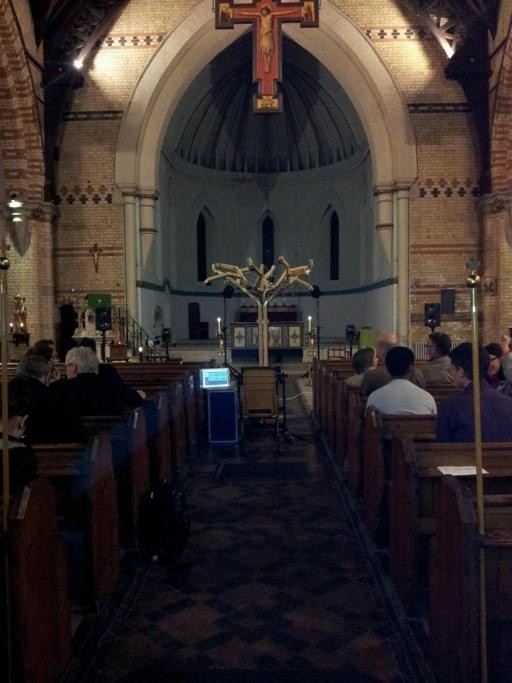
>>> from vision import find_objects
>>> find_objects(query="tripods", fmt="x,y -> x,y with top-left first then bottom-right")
222,302 -> 241,377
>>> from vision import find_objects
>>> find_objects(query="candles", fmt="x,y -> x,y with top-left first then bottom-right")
217,317 -> 222,335
308,315 -> 312,332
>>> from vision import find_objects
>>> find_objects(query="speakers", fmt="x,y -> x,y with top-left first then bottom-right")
162,327 -> 171,344
424,303 -> 441,328
345,324 -> 356,339
441,289 -> 455,311
96,306 -> 113,329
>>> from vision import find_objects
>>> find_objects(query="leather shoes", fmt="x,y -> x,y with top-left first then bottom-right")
117,549 -> 159,577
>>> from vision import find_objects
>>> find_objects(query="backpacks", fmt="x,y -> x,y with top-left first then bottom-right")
138,477 -> 192,564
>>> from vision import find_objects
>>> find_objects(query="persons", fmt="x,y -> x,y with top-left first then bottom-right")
345,330 -> 512,495
236,5 -> 300,74
1,330 -> 151,504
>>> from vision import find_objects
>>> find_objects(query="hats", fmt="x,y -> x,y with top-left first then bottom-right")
351,346 -> 377,376
